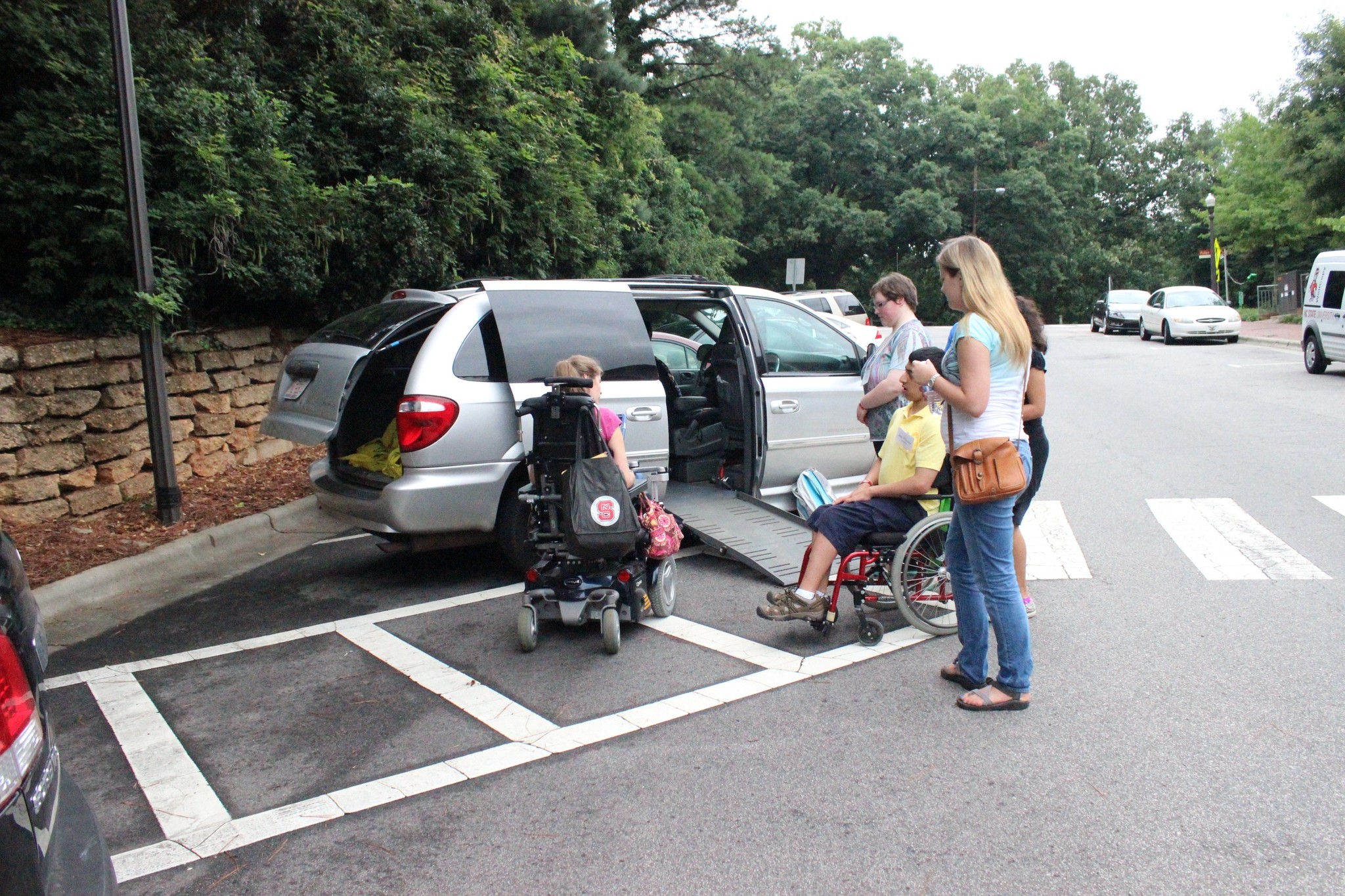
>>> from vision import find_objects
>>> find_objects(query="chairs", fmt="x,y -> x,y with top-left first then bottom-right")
653,356 -> 707,409
709,359 -> 745,465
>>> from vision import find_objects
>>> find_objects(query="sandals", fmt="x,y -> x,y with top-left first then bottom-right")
940,658 -> 986,691
956,677 -> 1029,711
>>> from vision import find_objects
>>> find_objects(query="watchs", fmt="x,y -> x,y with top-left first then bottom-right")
928,373 -> 941,387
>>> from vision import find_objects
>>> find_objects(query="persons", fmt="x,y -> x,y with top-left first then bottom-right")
529,354 -> 642,490
914,236 -> 1033,710
983,296 -> 1048,622
757,347 -> 949,621
856,274 -> 932,455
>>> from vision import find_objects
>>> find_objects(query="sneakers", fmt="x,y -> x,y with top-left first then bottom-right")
766,586 -> 830,619
756,590 -> 823,621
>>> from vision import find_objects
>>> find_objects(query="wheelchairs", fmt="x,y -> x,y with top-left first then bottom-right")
802,493 -> 959,647
513,375 -> 678,655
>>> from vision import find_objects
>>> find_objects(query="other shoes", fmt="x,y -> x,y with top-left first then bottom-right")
988,600 -> 1036,622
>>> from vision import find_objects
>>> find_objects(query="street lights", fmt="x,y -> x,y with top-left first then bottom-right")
1205,192 -> 1218,293
972,165 -> 1006,237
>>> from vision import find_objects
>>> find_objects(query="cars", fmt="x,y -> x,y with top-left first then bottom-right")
1138,285 -> 1241,345
0,532 -> 120,896
1090,289 -> 1152,335
765,311 -> 893,353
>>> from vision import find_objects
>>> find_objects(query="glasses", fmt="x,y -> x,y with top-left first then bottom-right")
873,298 -> 892,310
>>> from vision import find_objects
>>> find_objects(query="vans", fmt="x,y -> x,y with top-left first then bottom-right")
1300,249 -> 1345,374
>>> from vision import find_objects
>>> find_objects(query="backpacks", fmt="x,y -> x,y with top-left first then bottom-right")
791,468 -> 838,521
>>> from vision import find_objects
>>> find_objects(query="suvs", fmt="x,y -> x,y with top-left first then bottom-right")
762,289 -> 871,326
258,277 -> 877,582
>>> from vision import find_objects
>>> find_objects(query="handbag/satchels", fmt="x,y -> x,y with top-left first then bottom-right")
559,406 -> 641,558
637,491 -> 683,558
953,436 -> 1025,503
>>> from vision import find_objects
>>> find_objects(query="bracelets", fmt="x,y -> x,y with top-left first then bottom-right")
859,403 -> 868,410
